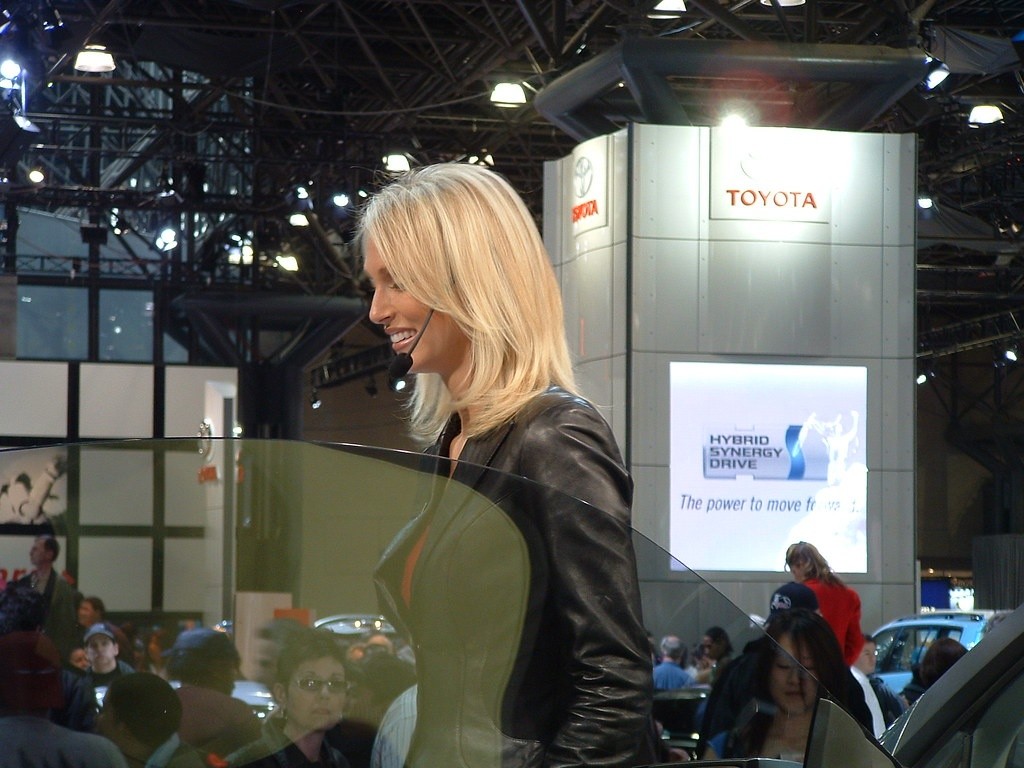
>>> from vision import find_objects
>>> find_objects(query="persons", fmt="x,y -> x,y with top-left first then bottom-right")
786,542 -> 863,666
696,582 -> 821,757
851,635 -> 905,738
360,163 -> 655,768
649,626 -> 734,735
707,607 -> 844,768
0,535 -> 417,768
921,638 -> 967,688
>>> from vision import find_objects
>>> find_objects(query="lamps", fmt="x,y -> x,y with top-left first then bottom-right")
490,58 -> 527,108
72,33 -> 116,73
969,105 -> 1005,129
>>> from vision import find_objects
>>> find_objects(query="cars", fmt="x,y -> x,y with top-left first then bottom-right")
1,434 -> 1023,768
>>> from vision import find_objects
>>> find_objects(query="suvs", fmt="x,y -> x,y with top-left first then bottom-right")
860,606 -> 1016,694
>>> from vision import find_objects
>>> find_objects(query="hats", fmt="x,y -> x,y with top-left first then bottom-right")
769,581 -> 820,614
0,630 -> 61,676
82,624 -> 115,643
159,626 -> 248,684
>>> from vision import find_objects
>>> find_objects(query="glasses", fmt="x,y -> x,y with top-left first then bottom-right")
290,677 -> 348,694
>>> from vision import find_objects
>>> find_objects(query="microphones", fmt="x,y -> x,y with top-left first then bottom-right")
389,309 -> 434,379
